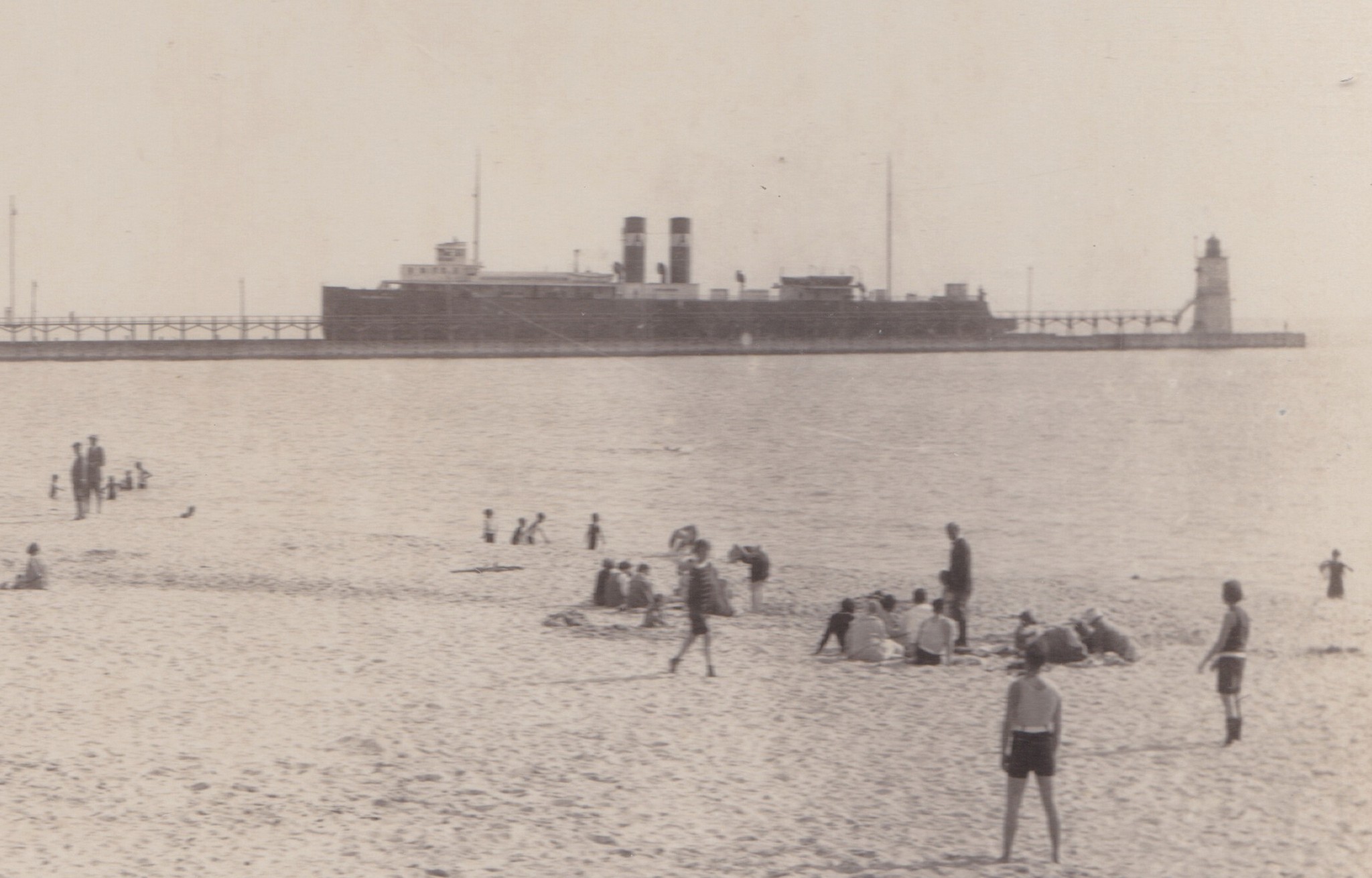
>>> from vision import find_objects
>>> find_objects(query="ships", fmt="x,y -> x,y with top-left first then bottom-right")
320,140 -> 1018,343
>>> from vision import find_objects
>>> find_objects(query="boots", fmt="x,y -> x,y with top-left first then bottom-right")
1225,717 -> 1241,744
707,665 -> 717,677
671,656 -> 684,673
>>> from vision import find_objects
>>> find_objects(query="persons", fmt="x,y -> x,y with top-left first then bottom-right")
1318,549 -> 1353,600
478,492 -> 1134,674
995,647 -> 1069,867
47,429 -> 156,517
2,540 -> 48,590
1196,579 -> 1252,745
